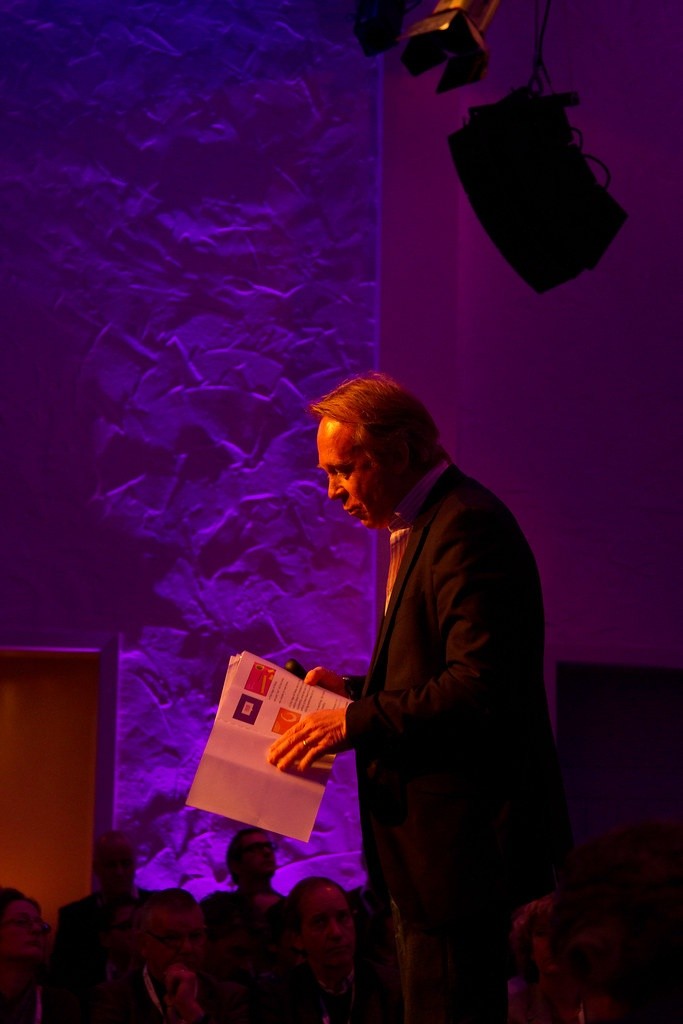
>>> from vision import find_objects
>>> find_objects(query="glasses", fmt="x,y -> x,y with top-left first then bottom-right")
3,917 -> 51,932
302,912 -> 356,925
240,843 -> 277,851
147,931 -> 206,944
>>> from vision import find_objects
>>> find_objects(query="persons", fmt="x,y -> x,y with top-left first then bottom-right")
0,878 -> 683,1024
267,372 -> 578,1024
51,832 -> 168,1010
599,817 -> 683,908
200,827 -> 292,959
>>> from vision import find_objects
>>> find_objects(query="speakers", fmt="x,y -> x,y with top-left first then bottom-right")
448,85 -> 628,295
